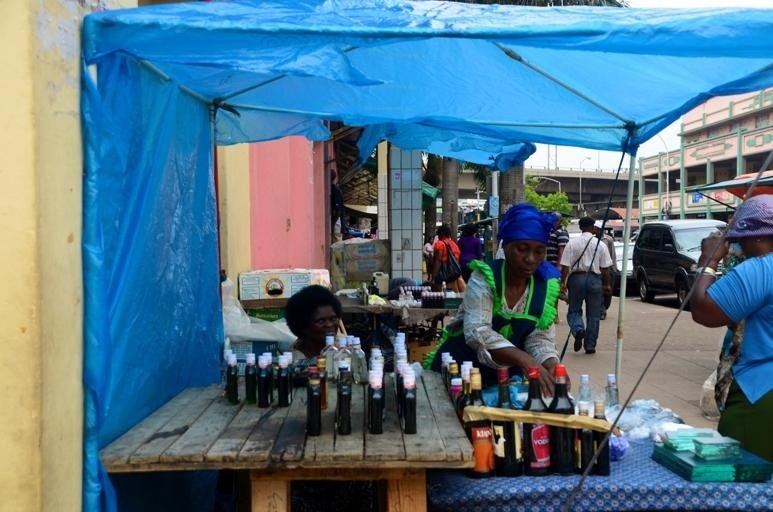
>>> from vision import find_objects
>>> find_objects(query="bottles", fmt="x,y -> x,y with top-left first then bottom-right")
439,351 -> 619,477
222,331 -> 418,436
403,283 -> 466,308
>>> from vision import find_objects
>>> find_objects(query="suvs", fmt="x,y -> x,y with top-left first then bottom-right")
632,218 -> 741,307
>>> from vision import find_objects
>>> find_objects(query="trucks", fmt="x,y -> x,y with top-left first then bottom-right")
562,217 -> 592,245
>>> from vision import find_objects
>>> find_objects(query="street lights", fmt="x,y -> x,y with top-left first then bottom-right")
532,176 -> 562,192
579,157 -> 592,208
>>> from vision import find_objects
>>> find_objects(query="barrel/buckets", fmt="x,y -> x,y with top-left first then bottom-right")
371,272 -> 389,297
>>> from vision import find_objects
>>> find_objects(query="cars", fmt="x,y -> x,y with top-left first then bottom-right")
609,240 -> 638,295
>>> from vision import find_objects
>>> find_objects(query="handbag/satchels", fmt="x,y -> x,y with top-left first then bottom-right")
433,239 -> 462,285
698,352 -> 738,421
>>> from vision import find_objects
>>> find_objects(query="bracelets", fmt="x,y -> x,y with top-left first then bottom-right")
696,267 -> 717,277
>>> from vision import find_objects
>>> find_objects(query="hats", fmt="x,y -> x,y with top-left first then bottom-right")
578,217 -> 595,226
721,192 -> 773,241
496,202 -> 558,248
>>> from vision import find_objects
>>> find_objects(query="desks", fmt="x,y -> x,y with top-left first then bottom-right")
390,302 -> 457,325
239,297 -> 392,347
99,369 -> 476,512
427,400 -> 773,512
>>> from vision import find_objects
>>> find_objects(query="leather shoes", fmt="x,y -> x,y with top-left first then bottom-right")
585,349 -> 596,354
573,329 -> 586,353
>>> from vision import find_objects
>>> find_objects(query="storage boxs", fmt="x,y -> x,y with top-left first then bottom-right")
231,342 -> 277,377
344,240 -> 390,281
239,268 -> 328,300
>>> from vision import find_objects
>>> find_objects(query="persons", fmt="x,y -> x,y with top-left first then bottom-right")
559,217 -> 613,353
285,285 -> 346,363
547,211 -> 569,324
691,193 -> 773,461
592,221 -> 617,320
421,225 -> 484,292
330,168 -> 344,243
422,203 -> 571,398
